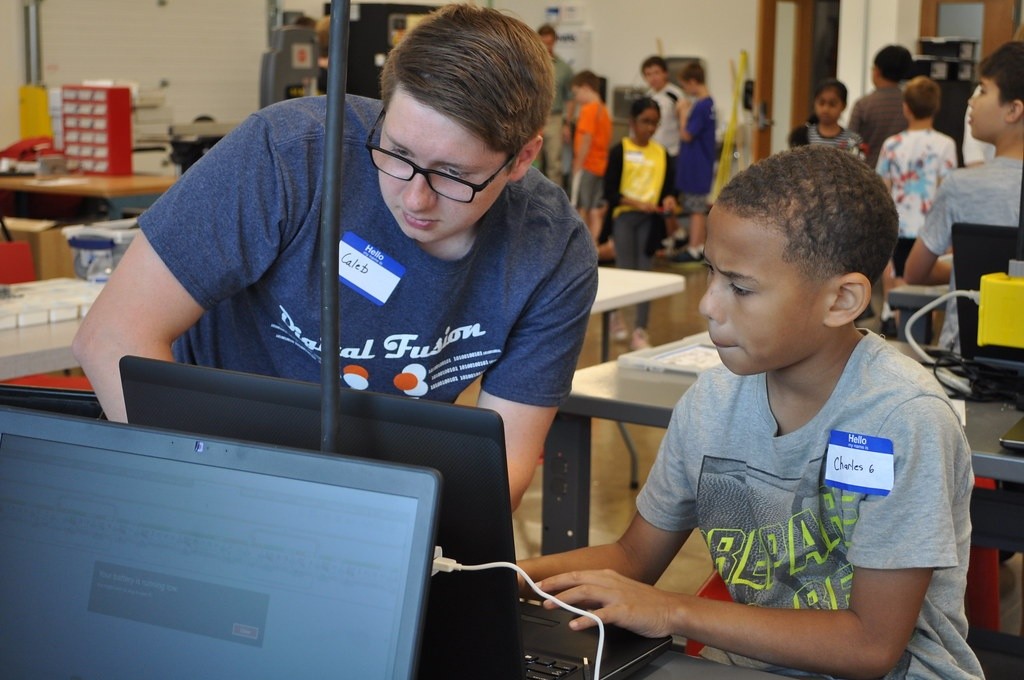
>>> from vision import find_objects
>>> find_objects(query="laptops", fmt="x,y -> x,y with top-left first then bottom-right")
119,355 -> 674,680
0,404 -> 443,680
951,220 -> 1024,378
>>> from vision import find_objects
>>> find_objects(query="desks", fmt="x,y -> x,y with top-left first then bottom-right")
0,175 -> 178,222
543,332 -> 1023,659
887,285 -> 949,345
590,266 -> 686,490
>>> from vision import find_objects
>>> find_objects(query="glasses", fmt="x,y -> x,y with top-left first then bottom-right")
633,118 -> 661,128
365,106 -> 520,204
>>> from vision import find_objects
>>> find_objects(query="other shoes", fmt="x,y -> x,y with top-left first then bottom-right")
669,248 -> 706,269
610,312 -> 628,343
630,330 -> 651,351
671,235 -> 689,249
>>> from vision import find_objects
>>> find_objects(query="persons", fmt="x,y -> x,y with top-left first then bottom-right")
72,3 -> 600,514
524,22 -> 718,355
517,143 -> 987,680
787,40 -> 1024,354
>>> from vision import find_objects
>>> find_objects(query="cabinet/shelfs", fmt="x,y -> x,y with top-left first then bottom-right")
61,85 -> 131,178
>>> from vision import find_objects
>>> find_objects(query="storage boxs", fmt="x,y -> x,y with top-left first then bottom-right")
0,215 -> 74,281
